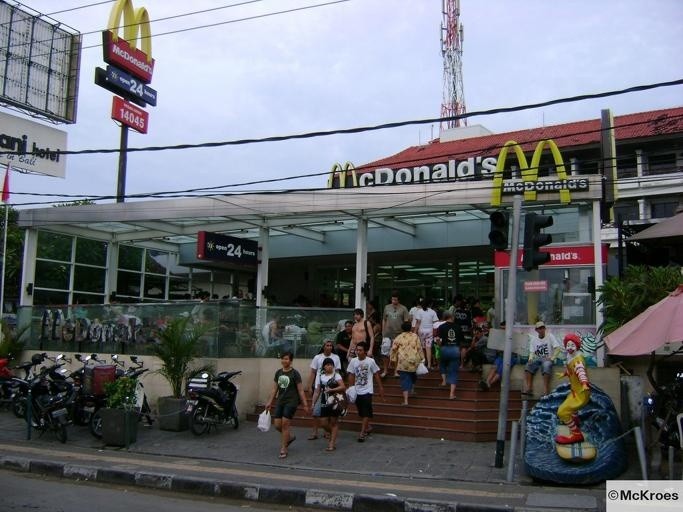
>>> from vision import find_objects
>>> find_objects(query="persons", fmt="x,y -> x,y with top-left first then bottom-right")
520,320 -> 560,396
480,321 -> 517,391
555,333 -> 591,444
71,289 -> 497,460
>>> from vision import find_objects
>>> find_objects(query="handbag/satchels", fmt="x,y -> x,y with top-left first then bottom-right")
367,337 -> 377,353
311,393 -> 322,416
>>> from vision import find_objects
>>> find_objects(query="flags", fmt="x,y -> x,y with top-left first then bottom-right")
1,162 -> 12,202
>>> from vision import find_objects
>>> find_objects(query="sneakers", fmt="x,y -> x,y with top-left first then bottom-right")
480,379 -> 490,391
522,389 -> 533,396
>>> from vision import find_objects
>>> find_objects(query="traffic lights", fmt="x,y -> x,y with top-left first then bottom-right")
487,210 -> 509,251
521,212 -> 554,271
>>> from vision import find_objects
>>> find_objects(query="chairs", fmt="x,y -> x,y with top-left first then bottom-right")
211,318 -> 334,358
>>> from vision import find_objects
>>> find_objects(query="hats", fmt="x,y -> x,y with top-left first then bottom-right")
535,321 -> 545,329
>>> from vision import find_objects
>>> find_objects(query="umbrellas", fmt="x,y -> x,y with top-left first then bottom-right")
623,205 -> 683,241
603,283 -> 683,356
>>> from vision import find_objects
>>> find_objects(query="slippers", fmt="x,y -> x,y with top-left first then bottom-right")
287,436 -> 296,446
279,451 -> 288,458
326,447 -> 336,451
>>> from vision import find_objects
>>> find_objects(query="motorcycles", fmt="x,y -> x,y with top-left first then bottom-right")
184,368 -> 243,438
0,350 -> 154,443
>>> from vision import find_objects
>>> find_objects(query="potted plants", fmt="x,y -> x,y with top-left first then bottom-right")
99,377 -> 141,445
139,313 -> 211,433
